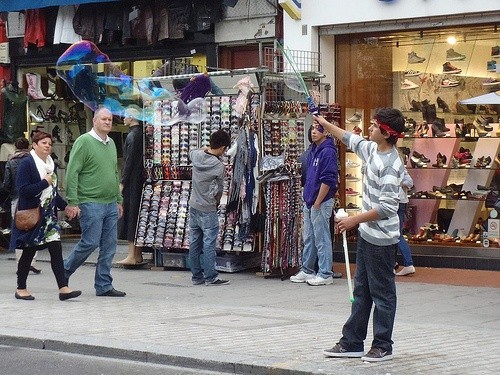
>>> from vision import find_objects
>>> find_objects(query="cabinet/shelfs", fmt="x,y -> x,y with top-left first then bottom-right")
346,107 -> 364,211
400,56 -> 500,249
29,97 -> 86,232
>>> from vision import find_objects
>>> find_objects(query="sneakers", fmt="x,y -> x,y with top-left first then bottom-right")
360,346 -> 393,362
441,49 -> 466,88
306,275 -> 334,286
323,342 -> 364,357
289,270 -> 316,283
483,45 -> 500,86
396,264 -> 415,276
193,278 -> 204,285
204,275 -> 231,287
400,52 -> 425,90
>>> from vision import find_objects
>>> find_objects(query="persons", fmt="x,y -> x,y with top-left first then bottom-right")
9,132 -> 82,300
188,130 -> 231,285
116,104 -> 143,264
63,107 -> 126,297
313,108 -> 415,362
290,121 -> 338,286
0,134 -> 29,249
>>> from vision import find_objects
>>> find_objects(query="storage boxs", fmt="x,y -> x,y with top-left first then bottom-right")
160,252 -> 186,268
199,253 -> 262,273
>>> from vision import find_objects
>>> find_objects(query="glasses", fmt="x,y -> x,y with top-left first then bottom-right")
135,95 -> 252,252
263,101 -> 310,176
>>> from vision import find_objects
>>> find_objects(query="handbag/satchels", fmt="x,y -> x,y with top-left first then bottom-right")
15,206 -> 41,231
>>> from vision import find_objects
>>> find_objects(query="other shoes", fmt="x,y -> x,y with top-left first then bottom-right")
59,290 -> 82,302
402,215 -> 491,244
401,96 -> 500,138
345,112 -> 363,211
28,265 -> 42,275
24,69 -> 84,168
407,183 -> 500,200
15,291 -> 36,300
398,147 -> 500,169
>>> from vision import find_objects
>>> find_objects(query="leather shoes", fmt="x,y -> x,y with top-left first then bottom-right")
96,287 -> 126,297
63,276 -> 69,288
